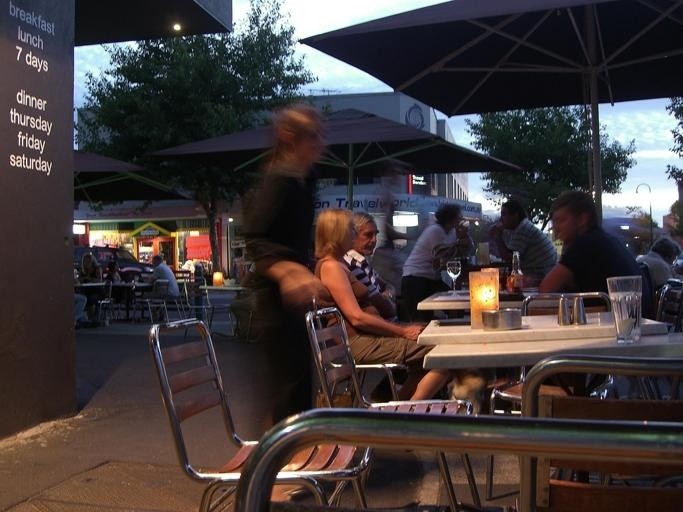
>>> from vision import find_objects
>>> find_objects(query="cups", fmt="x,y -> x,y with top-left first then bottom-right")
476,240 -> 490,265
605,275 -> 645,344
482,307 -> 521,331
213,271 -> 224,286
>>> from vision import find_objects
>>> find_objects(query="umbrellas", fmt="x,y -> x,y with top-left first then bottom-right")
297,0 -> 683,229
73,150 -> 192,202
143,108 -> 523,212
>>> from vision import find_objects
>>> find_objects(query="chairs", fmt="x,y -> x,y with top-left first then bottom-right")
518,354 -> 681,509
305,307 -> 479,509
647,278 -> 682,332
143,313 -> 371,511
74,248 -> 253,337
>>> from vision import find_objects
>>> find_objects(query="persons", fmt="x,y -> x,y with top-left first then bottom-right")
231,107 -> 330,442
75,295 -> 86,324
143,255 -> 180,322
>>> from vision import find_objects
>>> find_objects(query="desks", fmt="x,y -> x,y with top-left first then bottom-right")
419,328 -> 683,511
416,287 -> 576,305
418,314 -> 670,342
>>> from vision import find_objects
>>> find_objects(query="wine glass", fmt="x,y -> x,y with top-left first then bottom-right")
446,261 -> 462,297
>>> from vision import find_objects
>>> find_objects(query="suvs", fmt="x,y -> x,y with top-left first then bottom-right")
73,242 -> 154,284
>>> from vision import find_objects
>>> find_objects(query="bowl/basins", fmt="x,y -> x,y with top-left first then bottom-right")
223,279 -> 235,287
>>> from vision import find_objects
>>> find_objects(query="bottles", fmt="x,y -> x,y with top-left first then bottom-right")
504,251 -> 524,294
557,292 -> 586,325
140,247 -> 151,262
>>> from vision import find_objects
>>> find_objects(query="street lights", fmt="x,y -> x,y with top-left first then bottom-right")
225,217 -> 236,279
634,182 -> 652,245
